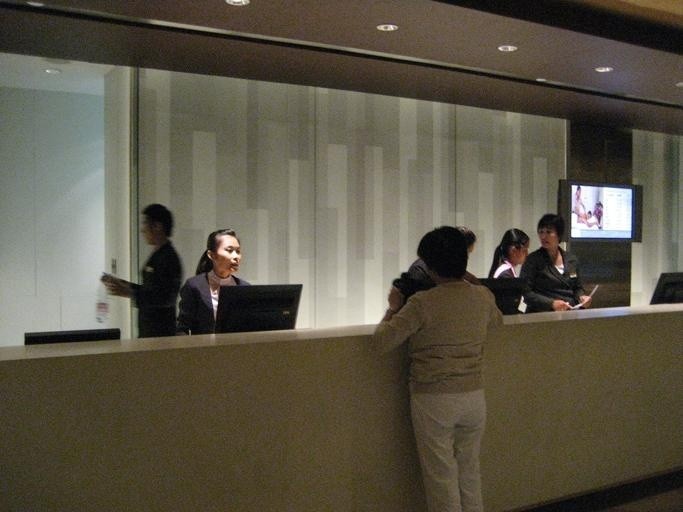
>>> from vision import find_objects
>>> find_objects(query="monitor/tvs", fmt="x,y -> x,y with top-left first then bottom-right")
479,275 -> 526,314
647,272 -> 683,306
24,327 -> 121,344
557,177 -> 644,246
214,283 -> 304,331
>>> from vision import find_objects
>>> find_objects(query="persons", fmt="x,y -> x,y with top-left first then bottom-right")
575,185 -> 604,229
371,223 -> 503,511
486,226 -> 531,315
381,226 -> 480,321
175,228 -> 253,337
518,212 -> 593,314
98,204 -> 181,336
